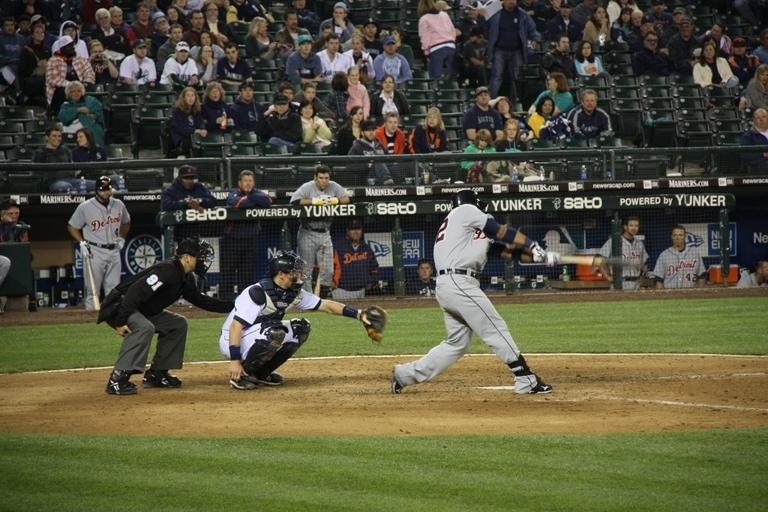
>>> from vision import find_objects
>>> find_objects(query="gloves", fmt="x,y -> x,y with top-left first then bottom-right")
528,238 -> 550,264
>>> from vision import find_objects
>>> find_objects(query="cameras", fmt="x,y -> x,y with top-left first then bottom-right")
90,52 -> 106,59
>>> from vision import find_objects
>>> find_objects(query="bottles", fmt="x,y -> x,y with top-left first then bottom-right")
538,165 -> 546,182
79,177 -> 86,192
405,163 -> 433,185
512,166 -> 518,183
550,171 -> 555,182
581,165 -> 587,179
221,111 -> 227,127
118,176 -> 125,189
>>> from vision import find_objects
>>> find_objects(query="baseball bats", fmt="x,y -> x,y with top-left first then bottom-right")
315,244 -> 326,296
87,257 -> 100,311
562,254 -> 637,271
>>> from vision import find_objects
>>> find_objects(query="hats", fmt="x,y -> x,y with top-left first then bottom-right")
1,199 -> 19,210
28,1 -> 748,133
178,165 -> 197,177
346,219 -> 363,230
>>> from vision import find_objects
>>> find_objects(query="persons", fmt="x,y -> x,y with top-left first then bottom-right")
95,236 -> 236,397
390,187 -> 554,395
1,1 -> 768,318
216,246 -> 389,390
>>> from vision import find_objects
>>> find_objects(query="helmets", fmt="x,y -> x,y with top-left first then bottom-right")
176,237 -> 215,278
452,189 -> 487,213
268,254 -> 308,285
95,176 -> 113,191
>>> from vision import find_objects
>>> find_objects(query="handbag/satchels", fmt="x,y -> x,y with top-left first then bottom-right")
550,118 -> 576,144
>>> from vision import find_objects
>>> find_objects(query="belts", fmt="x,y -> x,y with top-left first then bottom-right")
86,241 -> 115,250
439,267 -> 480,280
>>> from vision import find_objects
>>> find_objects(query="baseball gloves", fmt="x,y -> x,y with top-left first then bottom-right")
359,305 -> 386,342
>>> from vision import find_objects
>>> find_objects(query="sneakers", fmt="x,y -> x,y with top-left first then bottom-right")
229,376 -> 258,390
104,377 -> 138,395
392,365 -> 407,394
383,178 -> 395,186
28,302 -> 38,312
247,372 -> 284,386
142,369 -> 182,389
514,372 -> 553,395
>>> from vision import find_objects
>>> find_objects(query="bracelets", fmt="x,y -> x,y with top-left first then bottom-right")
229,346 -> 241,360
